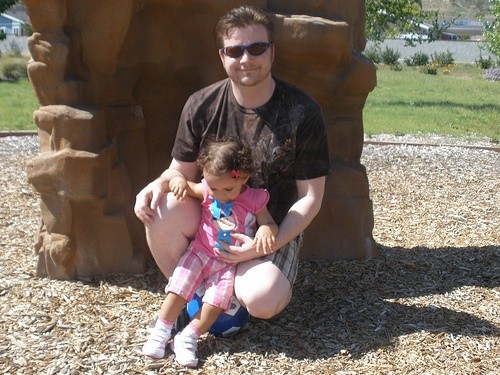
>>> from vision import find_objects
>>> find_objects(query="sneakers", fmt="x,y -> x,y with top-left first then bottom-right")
170,331 -> 199,368
142,323 -> 171,358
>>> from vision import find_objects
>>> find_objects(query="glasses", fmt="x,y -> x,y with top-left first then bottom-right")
217,40 -> 273,59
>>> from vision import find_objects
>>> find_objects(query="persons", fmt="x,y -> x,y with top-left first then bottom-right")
138,137 -> 284,368
133,3 -> 329,321
